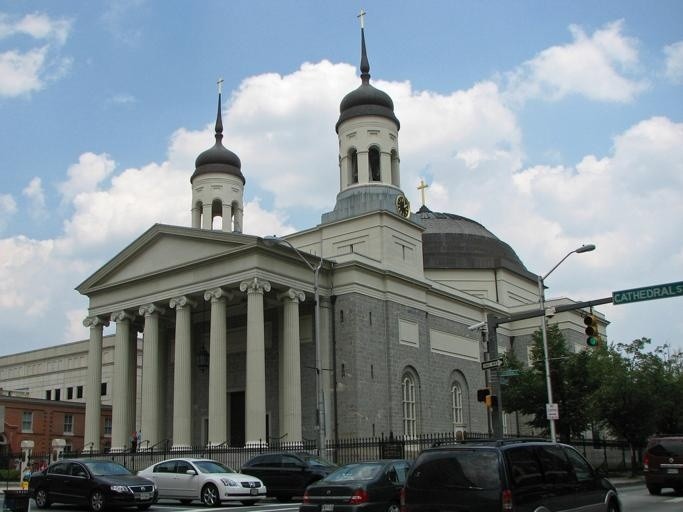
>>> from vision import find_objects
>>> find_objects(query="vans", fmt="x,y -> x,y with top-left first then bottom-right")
400,443 -> 625,512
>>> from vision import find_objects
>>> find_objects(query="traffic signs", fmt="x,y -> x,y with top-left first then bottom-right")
611,281 -> 682,304
481,357 -> 504,370
499,378 -> 511,387
496,369 -> 528,376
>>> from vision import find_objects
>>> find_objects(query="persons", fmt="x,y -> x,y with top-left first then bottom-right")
23,462 -> 33,481
138,429 -> 142,447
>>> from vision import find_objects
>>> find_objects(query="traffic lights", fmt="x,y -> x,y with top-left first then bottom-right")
486,395 -> 498,408
476,388 -> 490,403
583,313 -> 601,349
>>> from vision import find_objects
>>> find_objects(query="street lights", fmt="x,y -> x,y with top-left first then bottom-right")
535,244 -> 597,440
263,234 -> 329,458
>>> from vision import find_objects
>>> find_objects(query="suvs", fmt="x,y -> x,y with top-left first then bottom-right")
641,434 -> 683,494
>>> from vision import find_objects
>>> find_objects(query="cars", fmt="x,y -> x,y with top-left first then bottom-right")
24,459 -> 158,510
240,451 -> 340,505
137,455 -> 268,507
298,458 -> 414,512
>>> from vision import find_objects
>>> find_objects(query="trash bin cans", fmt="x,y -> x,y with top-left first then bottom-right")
4,490 -> 34,512
